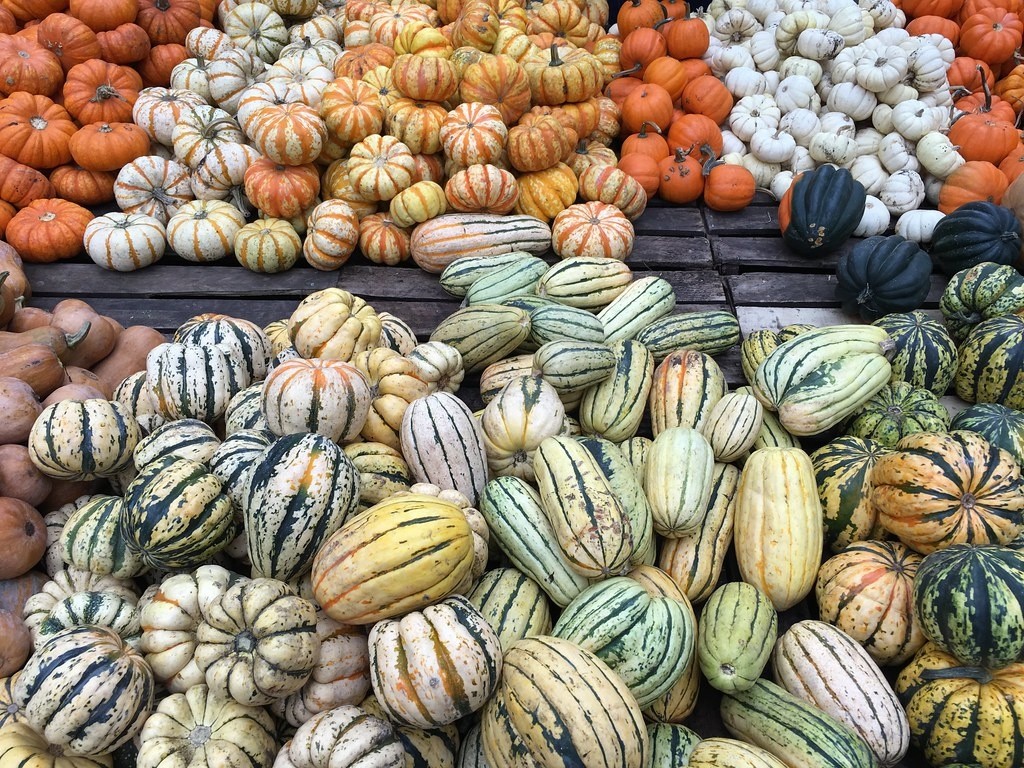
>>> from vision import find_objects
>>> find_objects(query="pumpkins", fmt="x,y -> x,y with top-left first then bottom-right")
0,0 -> 1024,768
